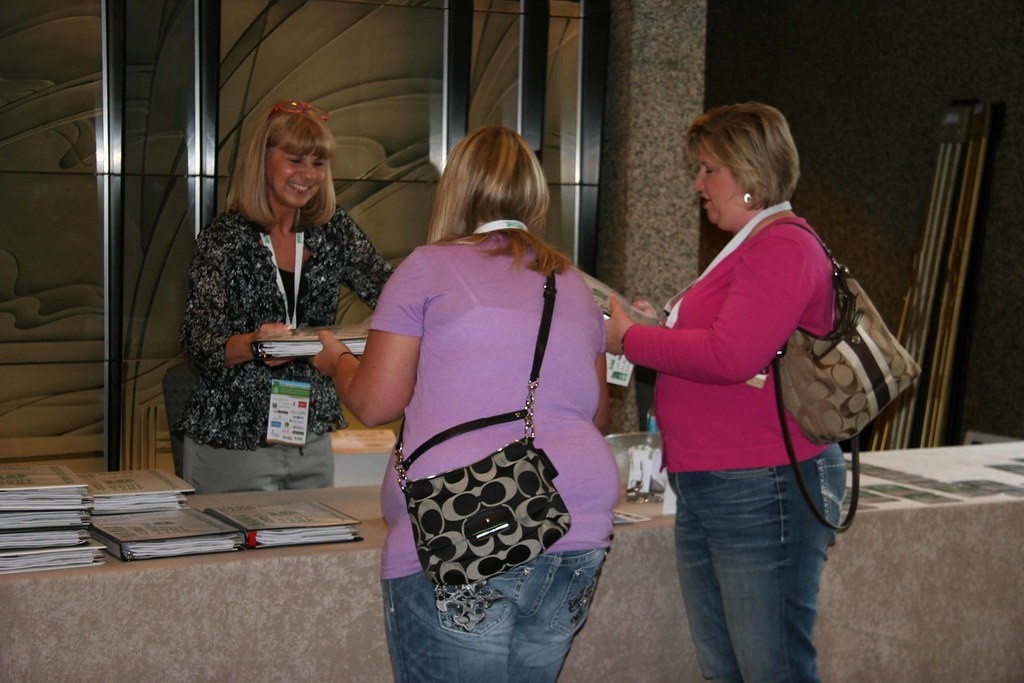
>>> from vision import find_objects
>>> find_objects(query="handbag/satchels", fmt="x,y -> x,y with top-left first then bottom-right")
403,438 -> 571,587
755,221 -> 922,444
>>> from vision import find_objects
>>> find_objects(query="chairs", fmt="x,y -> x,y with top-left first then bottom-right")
605,431 -> 662,482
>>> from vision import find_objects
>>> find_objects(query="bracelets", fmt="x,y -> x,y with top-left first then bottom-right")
338,350 -> 352,358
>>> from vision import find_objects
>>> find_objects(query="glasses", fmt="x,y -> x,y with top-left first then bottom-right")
270,99 -> 329,123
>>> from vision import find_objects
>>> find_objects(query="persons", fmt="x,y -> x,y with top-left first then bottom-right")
175,100 -> 397,491
604,103 -> 846,683
313,125 -> 621,683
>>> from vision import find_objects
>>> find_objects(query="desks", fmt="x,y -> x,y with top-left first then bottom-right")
0,441 -> 1024,683
